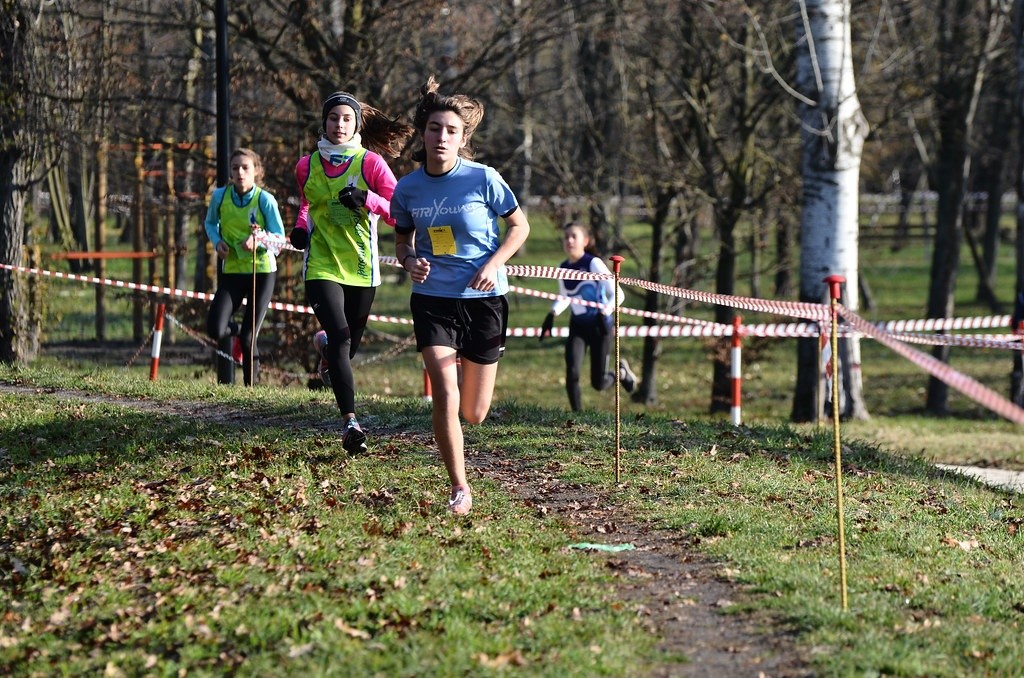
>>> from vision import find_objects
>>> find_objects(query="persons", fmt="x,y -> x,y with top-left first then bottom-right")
388,75 -> 530,521
539,222 -> 636,415
204,149 -> 286,386
290,92 -> 398,455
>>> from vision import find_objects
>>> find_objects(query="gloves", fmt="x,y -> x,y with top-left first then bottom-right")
596,314 -> 608,335
539,313 -> 554,342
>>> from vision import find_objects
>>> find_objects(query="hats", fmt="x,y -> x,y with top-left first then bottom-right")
323,91 -> 361,135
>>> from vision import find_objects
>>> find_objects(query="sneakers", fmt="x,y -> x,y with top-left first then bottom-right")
448,486 -> 473,516
619,358 -> 635,393
340,427 -> 368,455
314,330 -> 335,388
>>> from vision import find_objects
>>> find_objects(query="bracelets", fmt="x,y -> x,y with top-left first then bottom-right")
403,255 -> 415,272
